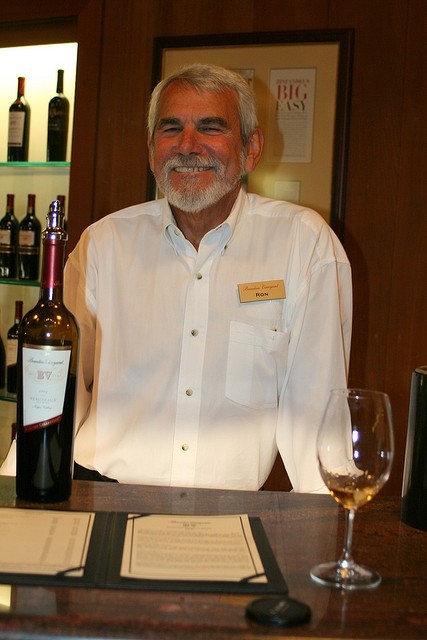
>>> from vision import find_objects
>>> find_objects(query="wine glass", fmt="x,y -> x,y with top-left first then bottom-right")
310,388 -> 394,593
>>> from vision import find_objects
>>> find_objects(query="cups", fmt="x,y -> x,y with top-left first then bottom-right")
398,367 -> 427,528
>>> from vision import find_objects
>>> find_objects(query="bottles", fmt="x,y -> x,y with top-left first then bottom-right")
6,300 -> 25,396
0,335 -> 5,389
17,199 -> 79,503
18,195 -> 39,277
56,194 -> 67,237
0,193 -> 18,278
47,69 -> 70,162
6,77 -> 30,162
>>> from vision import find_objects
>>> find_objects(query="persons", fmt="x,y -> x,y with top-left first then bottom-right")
0,62 -> 364,495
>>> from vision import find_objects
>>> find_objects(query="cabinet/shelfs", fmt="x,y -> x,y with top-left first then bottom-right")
0,3 -> 79,453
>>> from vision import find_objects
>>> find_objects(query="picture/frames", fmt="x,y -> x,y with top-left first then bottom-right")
145,27 -> 356,243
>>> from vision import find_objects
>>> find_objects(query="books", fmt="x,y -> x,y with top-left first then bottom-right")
0,505 -> 291,597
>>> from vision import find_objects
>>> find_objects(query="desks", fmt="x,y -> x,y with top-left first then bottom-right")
0,474 -> 425,635
